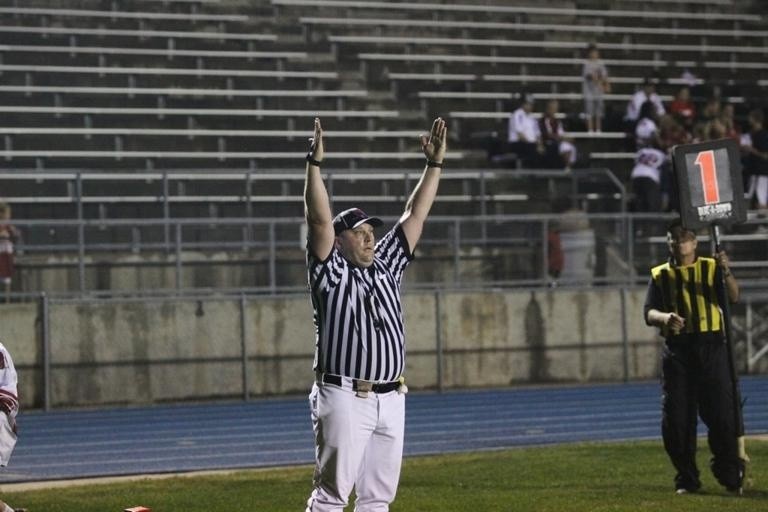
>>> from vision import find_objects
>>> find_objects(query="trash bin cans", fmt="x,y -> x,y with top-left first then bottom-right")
557,230 -> 596,285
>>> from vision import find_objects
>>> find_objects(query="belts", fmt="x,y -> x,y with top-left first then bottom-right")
323,373 -> 401,393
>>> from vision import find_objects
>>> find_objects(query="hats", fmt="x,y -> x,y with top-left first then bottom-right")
666,218 -> 696,237
332,208 -> 383,233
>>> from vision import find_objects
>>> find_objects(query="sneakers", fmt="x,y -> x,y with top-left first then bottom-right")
676,489 -> 691,494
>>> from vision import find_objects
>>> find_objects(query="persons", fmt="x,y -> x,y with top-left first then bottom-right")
582,44 -> 613,133
622,68 -> 768,211
0,342 -> 20,467
642,221 -> 748,495
0,202 -> 24,303
302,116 -> 450,512
505,93 -> 546,168
537,100 -> 578,172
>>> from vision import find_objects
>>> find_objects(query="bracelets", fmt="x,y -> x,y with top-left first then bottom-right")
426,160 -> 443,169
306,153 -> 322,166
723,271 -> 731,277
